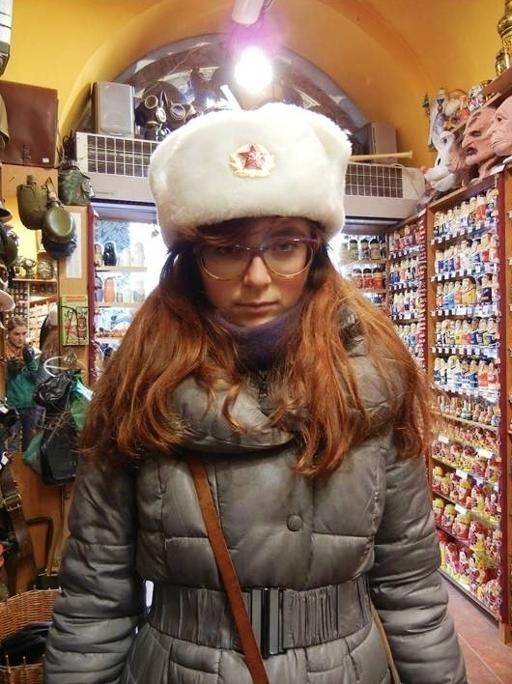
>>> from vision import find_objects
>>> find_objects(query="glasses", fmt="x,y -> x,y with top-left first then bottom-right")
190,229 -> 321,283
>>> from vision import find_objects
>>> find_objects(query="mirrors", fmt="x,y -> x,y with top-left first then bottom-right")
1,276 -> 63,453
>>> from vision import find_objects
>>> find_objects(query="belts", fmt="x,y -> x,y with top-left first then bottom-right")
0,456 -> 35,561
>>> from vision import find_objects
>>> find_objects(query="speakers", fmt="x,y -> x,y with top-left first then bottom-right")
91,80 -> 135,139
351,121 -> 399,164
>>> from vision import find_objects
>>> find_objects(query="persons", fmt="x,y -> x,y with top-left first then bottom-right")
461,105 -> 500,180
5,317 -> 34,362
42,101 -> 469,682
486,94 -> 512,160
5,309 -> 61,405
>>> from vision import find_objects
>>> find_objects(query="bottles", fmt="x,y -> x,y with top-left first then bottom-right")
17,159 -> 91,244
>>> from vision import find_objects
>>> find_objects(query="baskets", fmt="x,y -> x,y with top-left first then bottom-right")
0,584 -> 70,684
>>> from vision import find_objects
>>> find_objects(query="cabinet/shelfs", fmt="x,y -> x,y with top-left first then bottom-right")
343,224 -> 390,319
428,168 -> 510,645
391,204 -> 427,360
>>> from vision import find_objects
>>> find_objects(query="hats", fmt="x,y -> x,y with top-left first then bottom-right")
146,98 -> 354,249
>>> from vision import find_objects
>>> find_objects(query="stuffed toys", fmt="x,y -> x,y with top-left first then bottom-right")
415,189 -> 502,622
333,222 -> 415,358
